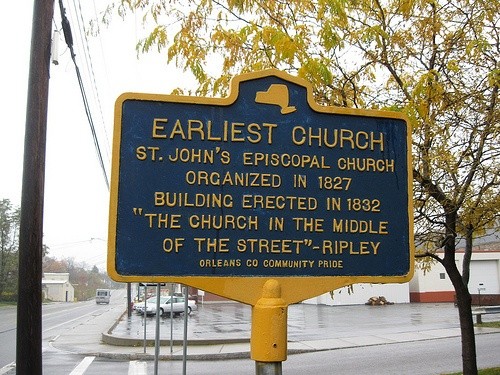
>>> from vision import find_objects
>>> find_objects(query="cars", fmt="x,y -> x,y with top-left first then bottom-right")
134,295 -> 197,317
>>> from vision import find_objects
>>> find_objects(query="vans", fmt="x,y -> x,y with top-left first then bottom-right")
96,289 -> 111,304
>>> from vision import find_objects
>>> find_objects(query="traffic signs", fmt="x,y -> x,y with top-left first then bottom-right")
105,68 -> 414,305
